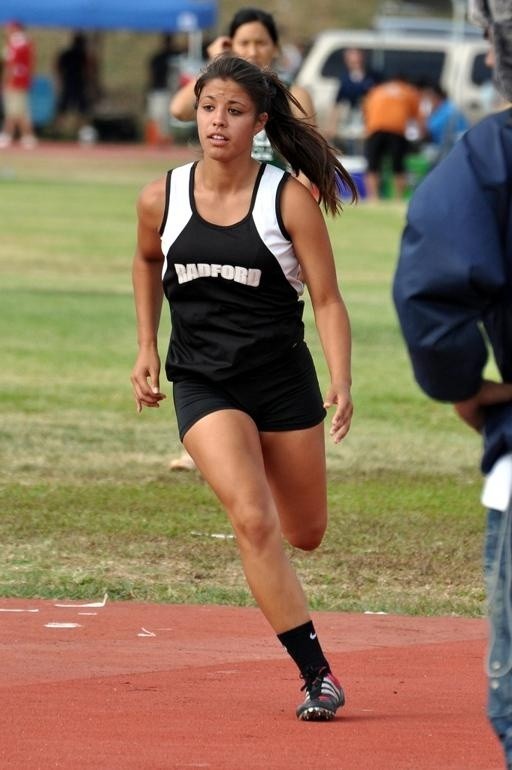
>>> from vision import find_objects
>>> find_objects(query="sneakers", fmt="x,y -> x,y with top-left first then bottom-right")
296,666 -> 345,721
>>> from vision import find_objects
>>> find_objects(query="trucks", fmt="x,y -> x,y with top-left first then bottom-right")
291,30 -> 497,174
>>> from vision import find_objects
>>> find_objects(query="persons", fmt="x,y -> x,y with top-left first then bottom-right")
131,55 -> 354,721
46,33 -> 99,142
270,46 -> 469,203
0,18 -> 36,149
165,8 -> 318,471
142,30 -> 180,142
392,0 -> 512,769
